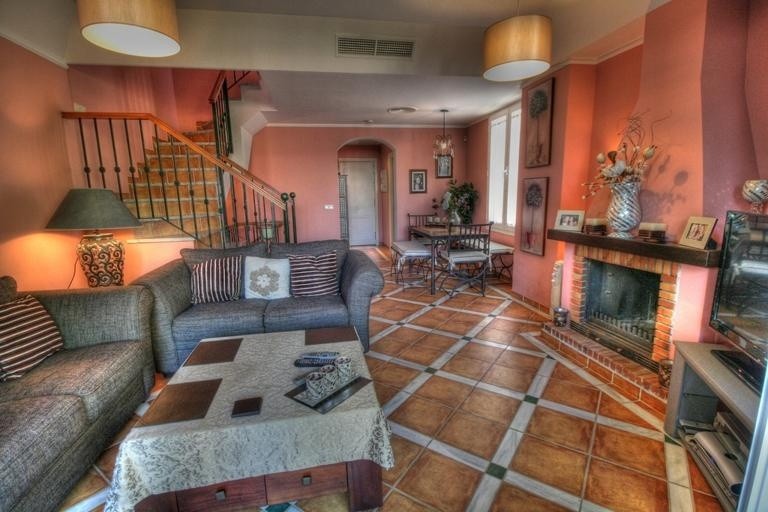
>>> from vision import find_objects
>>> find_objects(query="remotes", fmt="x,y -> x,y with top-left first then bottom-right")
301,351 -> 340,358
295,359 -> 338,367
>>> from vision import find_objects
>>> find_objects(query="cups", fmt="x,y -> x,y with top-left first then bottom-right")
307,373 -> 325,398
426,216 -> 448,225
553,307 -> 569,327
320,365 -> 337,390
334,356 -> 350,382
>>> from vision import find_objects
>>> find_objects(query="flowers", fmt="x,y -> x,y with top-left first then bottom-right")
432,192 -> 471,212
579,110 -> 670,199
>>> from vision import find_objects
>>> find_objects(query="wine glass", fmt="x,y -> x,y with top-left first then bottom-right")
742,179 -> 768,215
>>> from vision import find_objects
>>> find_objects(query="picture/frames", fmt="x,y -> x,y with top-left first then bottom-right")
520,178 -> 549,256
554,209 -> 585,232
678,216 -> 719,249
409,169 -> 428,193
435,155 -> 453,178
526,76 -> 555,168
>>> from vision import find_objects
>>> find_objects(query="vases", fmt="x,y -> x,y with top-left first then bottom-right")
447,208 -> 461,232
606,181 -> 642,239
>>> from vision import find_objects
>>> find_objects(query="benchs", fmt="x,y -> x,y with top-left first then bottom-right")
391,213 -> 513,295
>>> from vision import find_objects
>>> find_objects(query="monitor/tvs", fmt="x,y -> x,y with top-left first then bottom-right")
709,210 -> 768,398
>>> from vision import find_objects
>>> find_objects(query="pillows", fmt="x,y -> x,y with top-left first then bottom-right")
0,294 -> 63,381
288,250 -> 342,297
244,256 -> 291,300
190,254 -> 243,304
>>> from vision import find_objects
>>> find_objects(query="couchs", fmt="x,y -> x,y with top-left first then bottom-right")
0,276 -> 154,512
130,245 -> 383,378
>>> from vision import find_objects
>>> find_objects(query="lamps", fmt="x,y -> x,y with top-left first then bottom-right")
77,0 -> 182,58
481,0 -> 553,82
432,109 -> 456,159
44,188 -> 144,287
